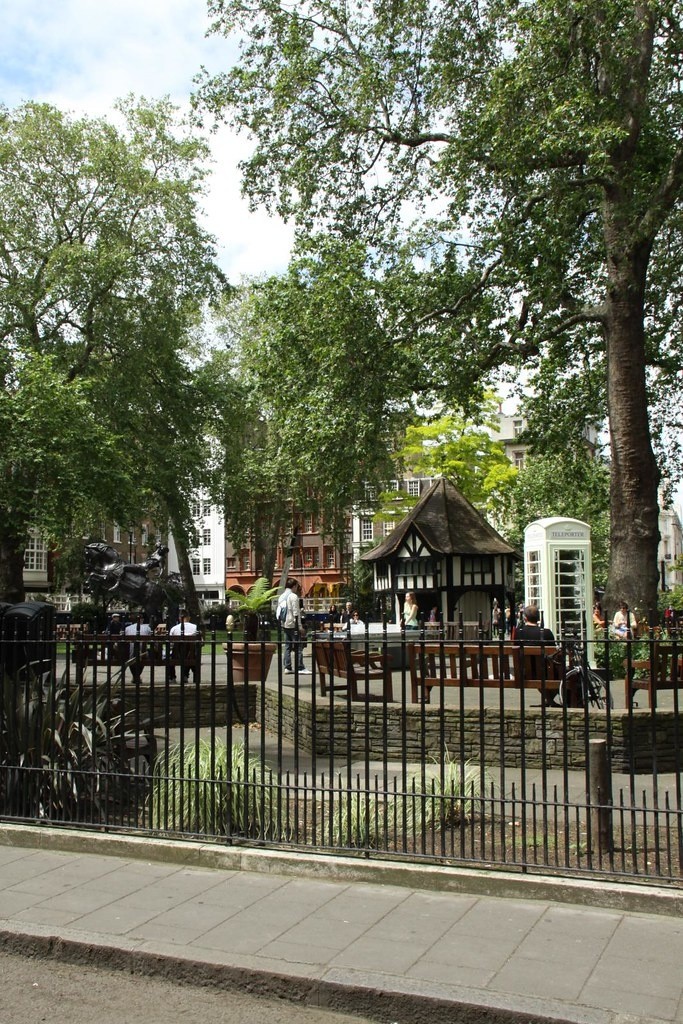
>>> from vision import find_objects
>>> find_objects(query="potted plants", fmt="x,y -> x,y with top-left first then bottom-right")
221,578 -> 282,683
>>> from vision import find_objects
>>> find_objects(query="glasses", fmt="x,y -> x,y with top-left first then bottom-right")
353,614 -> 359,617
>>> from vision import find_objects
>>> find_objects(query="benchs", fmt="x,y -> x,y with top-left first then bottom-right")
314,639 -> 393,702
72,634 -> 201,684
408,642 -> 579,709
623,641 -> 683,709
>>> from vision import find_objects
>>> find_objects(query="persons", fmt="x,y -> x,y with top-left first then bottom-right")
106,613 -> 125,654
398,592 -> 418,630
428,607 -> 437,631
277,579 -> 312,674
125,613 -> 153,684
592,605 -> 612,639
490,601 -> 525,636
612,602 -> 637,644
327,601 -> 365,632
167,609 -> 198,683
108,538 -> 169,592
514,605 -> 564,708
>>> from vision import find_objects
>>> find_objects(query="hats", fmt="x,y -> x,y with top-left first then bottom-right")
112,613 -> 120,619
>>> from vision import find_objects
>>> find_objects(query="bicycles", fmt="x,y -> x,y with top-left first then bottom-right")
549,629 -> 615,711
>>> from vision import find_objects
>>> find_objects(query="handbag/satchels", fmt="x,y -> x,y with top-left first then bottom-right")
276,591 -> 294,622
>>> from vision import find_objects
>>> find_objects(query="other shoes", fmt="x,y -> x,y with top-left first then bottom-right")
297,669 -> 312,675
284,668 -> 295,674
